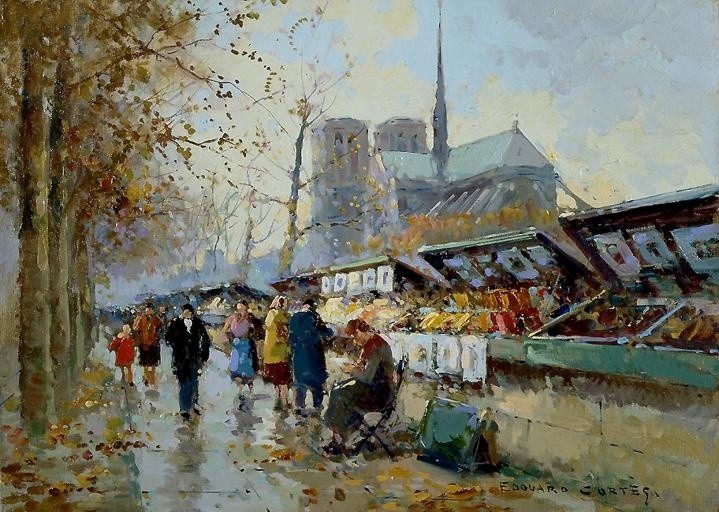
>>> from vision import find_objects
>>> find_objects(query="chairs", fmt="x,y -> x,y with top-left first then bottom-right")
350,351 -> 410,462
